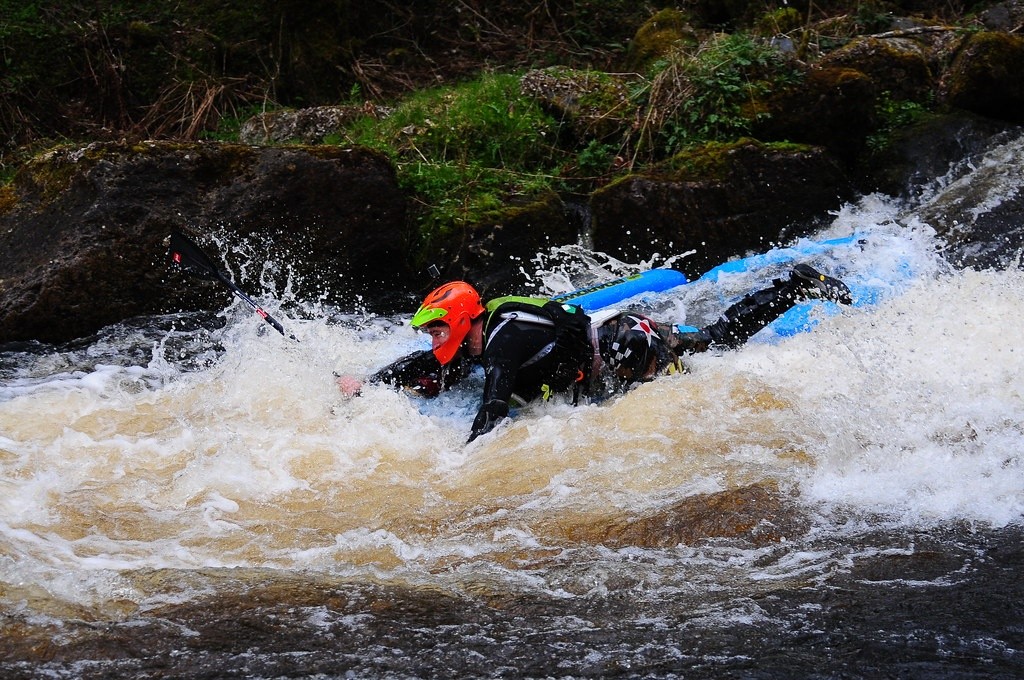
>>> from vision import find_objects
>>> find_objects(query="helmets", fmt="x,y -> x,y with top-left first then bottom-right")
408,281 -> 486,366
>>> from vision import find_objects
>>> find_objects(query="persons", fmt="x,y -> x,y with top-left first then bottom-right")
338,265 -> 853,445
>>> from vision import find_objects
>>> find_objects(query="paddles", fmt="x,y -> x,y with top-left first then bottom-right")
168,229 -> 363,397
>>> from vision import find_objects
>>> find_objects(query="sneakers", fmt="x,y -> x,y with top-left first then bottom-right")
788,264 -> 852,306
